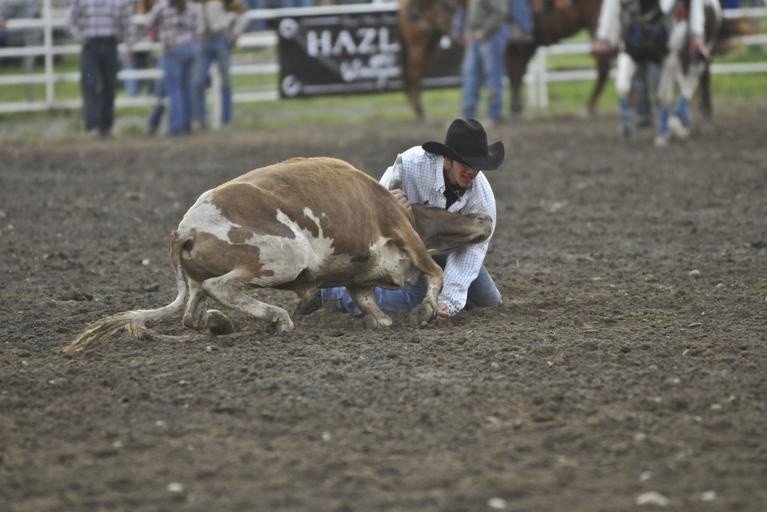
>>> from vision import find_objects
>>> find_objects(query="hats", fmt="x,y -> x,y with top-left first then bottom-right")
422,118 -> 504,170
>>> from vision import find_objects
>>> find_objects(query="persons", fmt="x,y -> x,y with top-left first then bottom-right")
67,1 -> 245,139
449,1 -> 534,129
592,1 -> 712,147
319,116 -> 505,319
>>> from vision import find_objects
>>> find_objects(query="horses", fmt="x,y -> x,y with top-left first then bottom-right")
618,2 -> 722,131
393,2 -> 611,124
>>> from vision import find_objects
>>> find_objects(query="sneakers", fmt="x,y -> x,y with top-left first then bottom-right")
292,283 -> 321,317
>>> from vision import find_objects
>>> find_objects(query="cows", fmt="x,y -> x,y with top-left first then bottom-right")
64,156 -> 495,354
